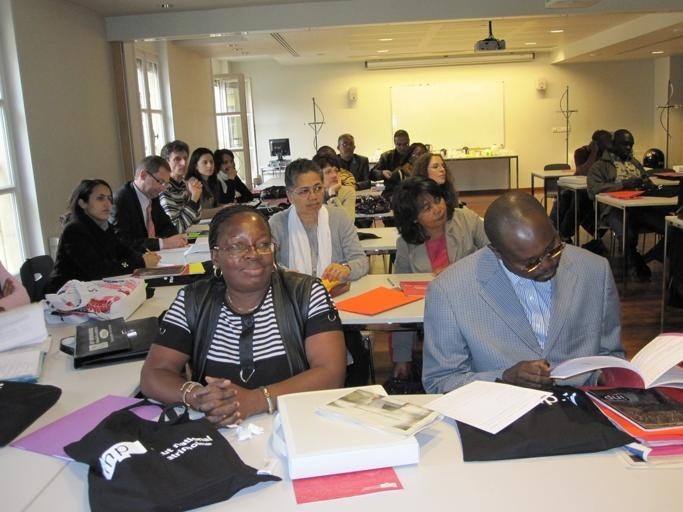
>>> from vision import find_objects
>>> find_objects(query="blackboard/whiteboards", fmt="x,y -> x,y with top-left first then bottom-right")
390,79 -> 505,151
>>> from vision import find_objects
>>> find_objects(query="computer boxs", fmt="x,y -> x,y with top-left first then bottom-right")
268,161 -> 291,170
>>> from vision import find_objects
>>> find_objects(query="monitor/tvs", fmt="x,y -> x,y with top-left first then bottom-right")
269,138 -> 291,161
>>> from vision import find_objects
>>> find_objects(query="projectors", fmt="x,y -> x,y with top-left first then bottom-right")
474,38 -> 506,51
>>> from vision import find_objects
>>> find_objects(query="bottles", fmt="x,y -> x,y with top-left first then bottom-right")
424,142 -> 511,157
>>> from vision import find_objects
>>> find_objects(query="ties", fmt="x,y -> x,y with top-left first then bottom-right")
146,205 -> 155,238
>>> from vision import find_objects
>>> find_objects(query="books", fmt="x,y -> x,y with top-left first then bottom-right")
549,332 -> 683,466
0,309 -> 52,383
131,218 -> 214,280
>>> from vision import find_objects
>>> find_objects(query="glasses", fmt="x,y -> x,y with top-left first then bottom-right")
146,171 -> 168,187
214,242 -> 273,257
525,243 -> 566,273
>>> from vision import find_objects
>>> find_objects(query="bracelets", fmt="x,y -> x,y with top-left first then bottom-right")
180,380 -> 204,407
342,264 -> 351,270
258,385 -> 275,415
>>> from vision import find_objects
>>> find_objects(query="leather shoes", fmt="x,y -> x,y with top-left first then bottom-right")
628,254 -> 651,281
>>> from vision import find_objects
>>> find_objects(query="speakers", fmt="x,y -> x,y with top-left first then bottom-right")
535,76 -> 546,91
348,87 -> 358,102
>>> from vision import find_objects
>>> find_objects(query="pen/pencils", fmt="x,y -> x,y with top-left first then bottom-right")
387,278 -> 395,286
146,248 -> 162,264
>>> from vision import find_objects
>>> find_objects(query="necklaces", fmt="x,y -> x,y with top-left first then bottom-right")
225,288 -> 259,312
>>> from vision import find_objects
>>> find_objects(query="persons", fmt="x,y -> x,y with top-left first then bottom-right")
311,134 -> 370,226
108,140 -> 252,253
371,129 -> 455,203
140,205 -> 347,428
0,261 -> 31,313
421,191 -> 627,394
390,176 -> 491,380
550,128 -> 683,308
267,159 -> 370,388
39,180 -> 161,300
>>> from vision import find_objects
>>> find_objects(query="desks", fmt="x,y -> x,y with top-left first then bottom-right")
0,163 -> 681,512
442,151 -> 518,192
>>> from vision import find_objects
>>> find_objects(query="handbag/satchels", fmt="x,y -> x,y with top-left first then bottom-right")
642,168 -> 680,197
41,279 -> 147,321
60,317 -> 160,369
355,196 -> 391,214
64,399 -> 281,512
260,186 -> 287,198
0,381 -> 61,447
144,260 -> 212,287
443,378 -> 641,461
